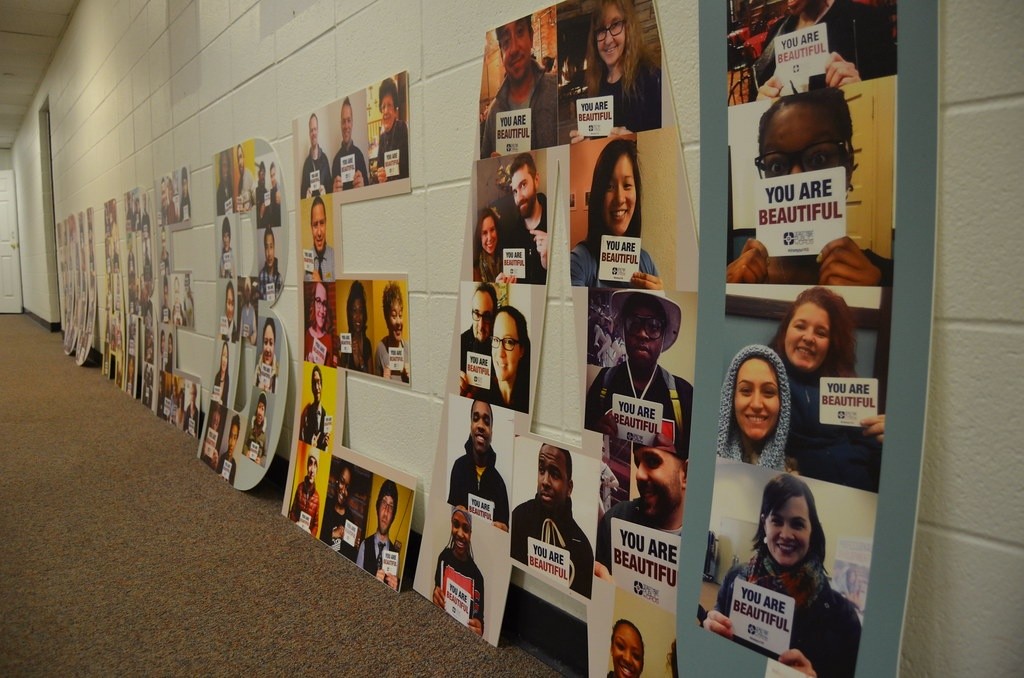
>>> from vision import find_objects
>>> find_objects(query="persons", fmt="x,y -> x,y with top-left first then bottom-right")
702,474 -> 860,678
303,280 -> 409,382
57,144 -> 284,485
457,284 -> 529,412
304,198 -> 335,281
473,152 -> 549,284
447,400 -> 509,533
748,0 -> 895,102
726,89 -> 893,285
718,288 -> 885,495
509,443 -> 594,597
285,450 -> 400,592
568,0 -> 662,144
300,366 -> 329,451
595,442 -> 686,583
584,289 -> 693,457
300,79 -> 408,199
432,505 -> 485,640
606,619 -> 645,678
569,138 -> 663,290
480,15 -> 559,160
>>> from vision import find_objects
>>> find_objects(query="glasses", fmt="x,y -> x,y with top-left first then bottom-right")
490,335 -> 518,351
471,309 -> 492,324
595,19 -> 626,42
757,141 -> 850,178
381,500 -> 395,511
621,313 -> 666,339
315,298 -> 329,312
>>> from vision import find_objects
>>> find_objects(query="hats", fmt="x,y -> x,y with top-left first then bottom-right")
258,391 -> 267,412
376,480 -> 400,521
307,447 -> 319,470
609,288 -> 681,352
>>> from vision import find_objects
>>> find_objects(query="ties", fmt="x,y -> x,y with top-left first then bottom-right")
376,542 -> 387,574
